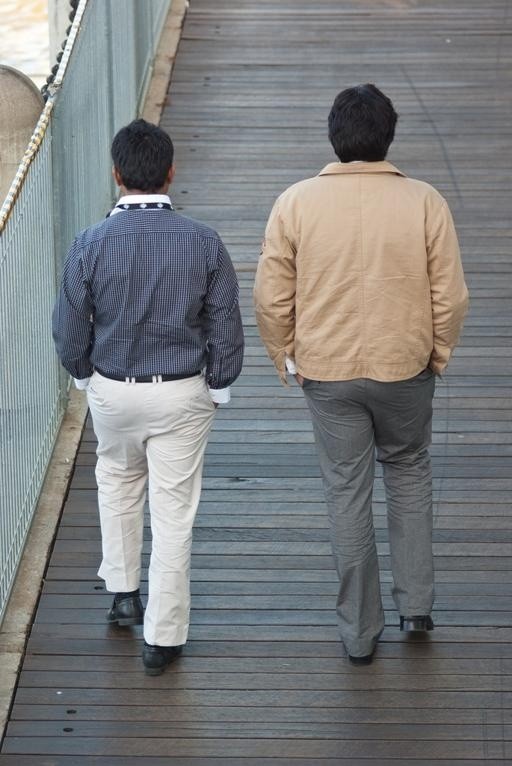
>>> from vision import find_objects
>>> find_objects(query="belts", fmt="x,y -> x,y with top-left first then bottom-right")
94,366 -> 203,384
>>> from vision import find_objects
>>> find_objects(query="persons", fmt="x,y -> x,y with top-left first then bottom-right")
252,83 -> 469,668
52,118 -> 245,676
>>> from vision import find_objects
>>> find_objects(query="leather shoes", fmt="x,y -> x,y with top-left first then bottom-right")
349,644 -> 376,666
399,614 -> 435,632
107,595 -> 144,628
142,643 -> 183,676
